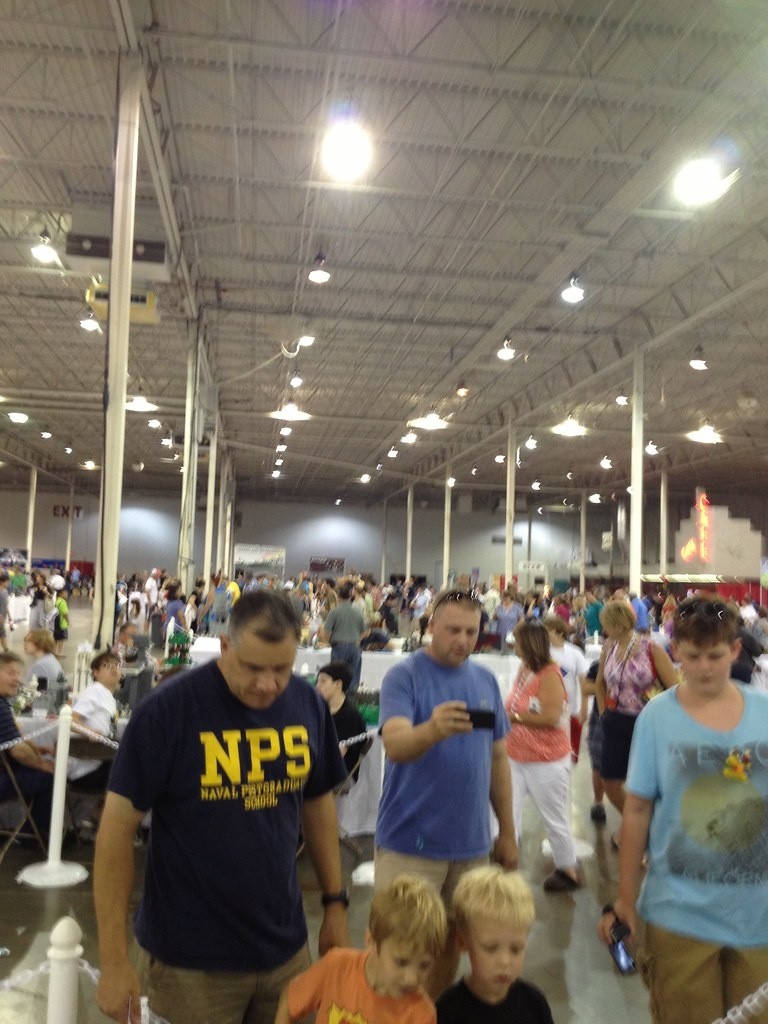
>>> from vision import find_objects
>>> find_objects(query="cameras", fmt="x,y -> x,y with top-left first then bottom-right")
609,924 -> 640,976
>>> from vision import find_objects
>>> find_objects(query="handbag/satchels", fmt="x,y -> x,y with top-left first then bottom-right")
642,640 -> 682,704
528,672 -> 571,731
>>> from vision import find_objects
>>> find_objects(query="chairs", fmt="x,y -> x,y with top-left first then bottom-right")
1,752 -> 46,861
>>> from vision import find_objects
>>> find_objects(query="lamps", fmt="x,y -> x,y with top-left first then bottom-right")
81,308 -> 97,328
615,389 -> 627,403
32,229 -> 54,261
562,276 -> 583,302
690,346 -> 706,370
39,369 -> 587,490
495,338 -> 515,360
309,250 -> 330,282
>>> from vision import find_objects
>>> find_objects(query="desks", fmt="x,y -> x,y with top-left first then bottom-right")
189,635 -> 522,700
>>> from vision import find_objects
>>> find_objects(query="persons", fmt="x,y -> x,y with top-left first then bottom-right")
434,866 -> 555,1024
92,590 -> 353,1024
597,595 -> 768,1023
0,567 -> 768,893
276,874 -> 448,1024
369,590 -> 522,997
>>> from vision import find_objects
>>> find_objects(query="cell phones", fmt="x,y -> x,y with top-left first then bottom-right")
455,708 -> 495,728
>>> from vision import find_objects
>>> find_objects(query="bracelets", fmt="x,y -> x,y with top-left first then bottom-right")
321,888 -> 350,907
515,711 -> 519,720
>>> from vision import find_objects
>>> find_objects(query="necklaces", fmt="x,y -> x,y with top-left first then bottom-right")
510,668 -> 534,712
605,631 -> 635,679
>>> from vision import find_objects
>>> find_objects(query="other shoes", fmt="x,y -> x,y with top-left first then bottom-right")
610,828 -> 623,852
590,806 -> 605,822
545,869 -> 580,891
133,834 -> 143,850
79,823 -> 97,842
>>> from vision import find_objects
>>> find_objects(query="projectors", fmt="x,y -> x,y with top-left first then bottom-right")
173,434 -> 210,450
67,231 -> 170,283
85,284 -> 163,322
